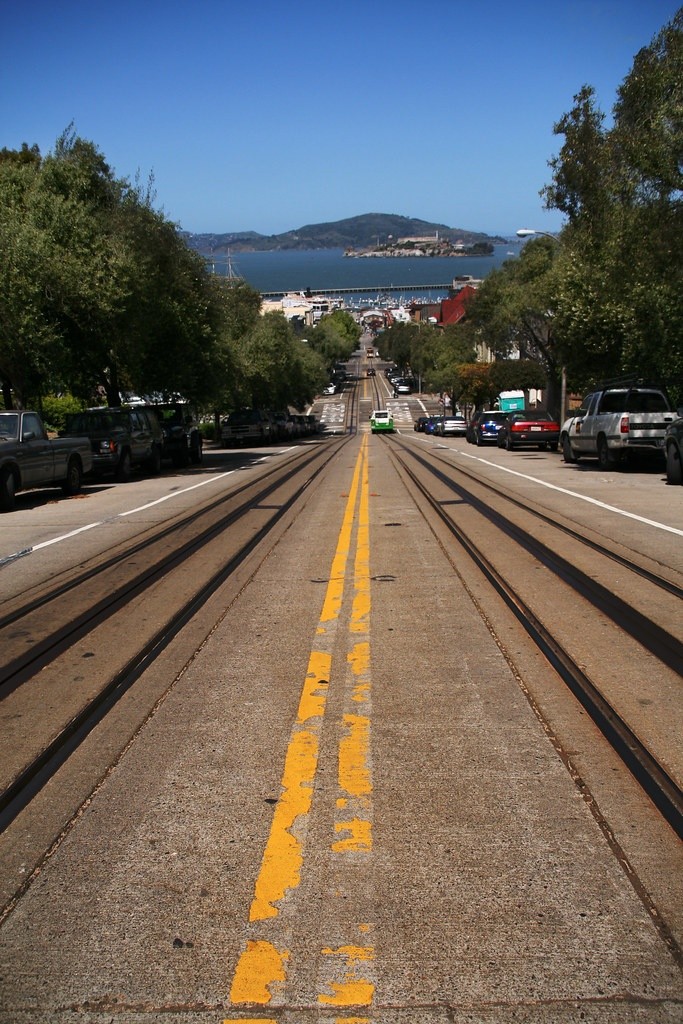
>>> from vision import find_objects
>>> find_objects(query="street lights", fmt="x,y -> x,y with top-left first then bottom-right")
516,229 -> 572,450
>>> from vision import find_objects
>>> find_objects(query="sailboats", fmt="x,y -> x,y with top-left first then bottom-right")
196,247 -> 246,292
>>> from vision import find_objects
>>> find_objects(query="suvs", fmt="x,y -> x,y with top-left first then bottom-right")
58,404 -> 203,483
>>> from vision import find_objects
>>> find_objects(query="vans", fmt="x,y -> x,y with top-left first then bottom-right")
369,410 -> 393,434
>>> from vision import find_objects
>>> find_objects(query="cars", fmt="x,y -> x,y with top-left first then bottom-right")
497,410 -> 559,451
322,383 -> 336,395
466,411 -> 513,447
367,369 -> 375,376
661,408 -> 683,484
414,414 -> 468,437
384,367 -> 411,394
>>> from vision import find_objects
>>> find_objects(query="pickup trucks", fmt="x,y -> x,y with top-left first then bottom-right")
220,406 -> 320,446
559,379 -> 682,471
0,410 -> 93,512
